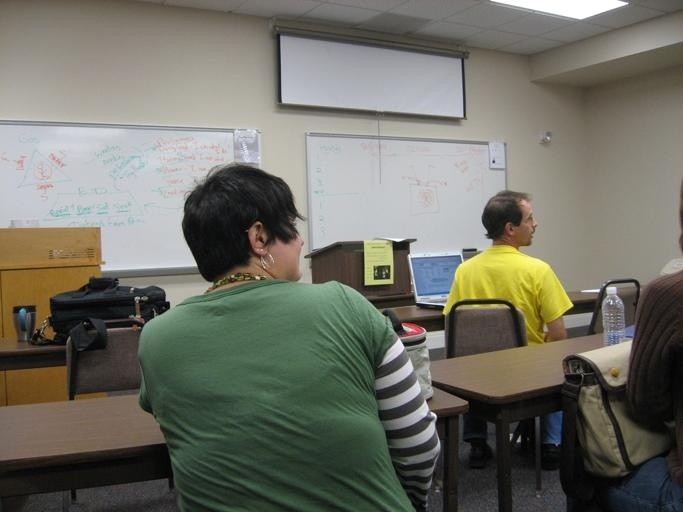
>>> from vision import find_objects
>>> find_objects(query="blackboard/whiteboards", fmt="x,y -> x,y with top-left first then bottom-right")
305,132 -> 508,303
0,119 -> 260,279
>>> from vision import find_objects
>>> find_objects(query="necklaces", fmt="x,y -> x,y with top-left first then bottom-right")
205,272 -> 266,293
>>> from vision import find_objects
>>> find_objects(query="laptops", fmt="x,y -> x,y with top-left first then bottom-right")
406,252 -> 464,309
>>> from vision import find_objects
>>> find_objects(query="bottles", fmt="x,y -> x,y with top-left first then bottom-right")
602,286 -> 625,344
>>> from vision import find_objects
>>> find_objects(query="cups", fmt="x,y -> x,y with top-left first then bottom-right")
11,305 -> 37,341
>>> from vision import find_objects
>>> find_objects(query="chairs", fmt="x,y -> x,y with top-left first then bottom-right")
64,316 -> 174,507
441,297 -> 544,499
586,277 -> 641,335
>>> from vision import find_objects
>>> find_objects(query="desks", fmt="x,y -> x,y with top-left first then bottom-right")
0,380 -> 468,510
422,324 -> 634,511
0,330 -> 66,373
374,283 -> 646,331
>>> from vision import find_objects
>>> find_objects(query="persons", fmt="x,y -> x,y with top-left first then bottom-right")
136,163 -> 440,512
567,183 -> 683,509
441,191 -> 574,471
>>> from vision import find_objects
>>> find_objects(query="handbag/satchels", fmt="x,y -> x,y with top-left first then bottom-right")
561,339 -> 668,480
30,277 -> 170,354
